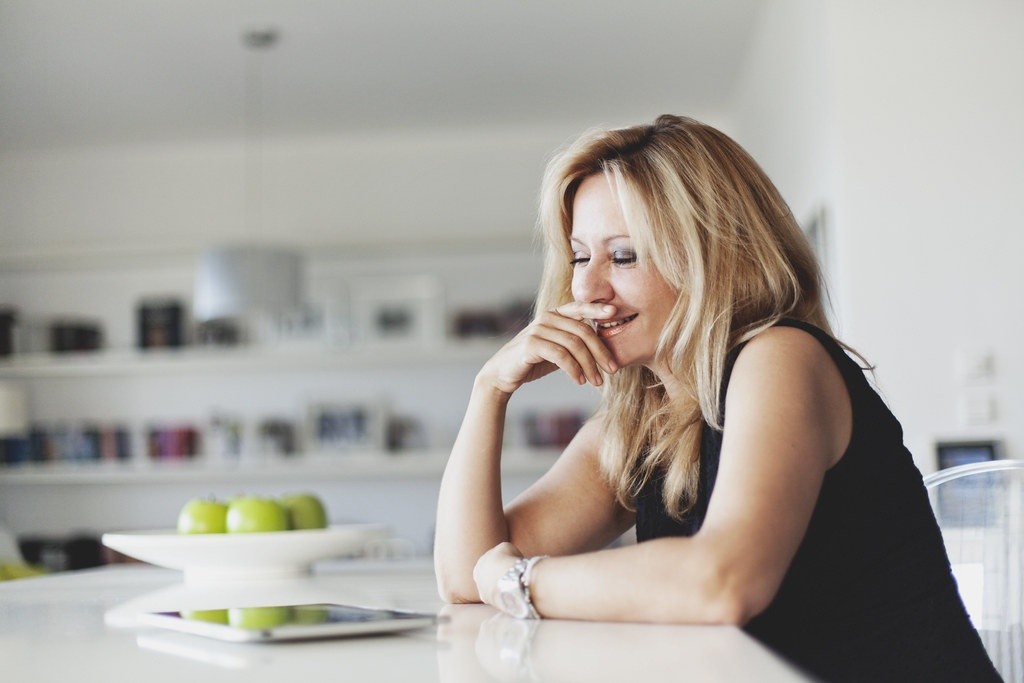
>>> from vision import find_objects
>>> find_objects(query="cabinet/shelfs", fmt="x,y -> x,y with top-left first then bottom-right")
0,241 -> 636,563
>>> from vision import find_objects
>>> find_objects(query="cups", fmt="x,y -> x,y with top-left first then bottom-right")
140,305 -> 180,349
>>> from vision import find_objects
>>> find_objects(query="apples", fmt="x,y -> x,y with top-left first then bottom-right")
175,608 -> 328,630
176,493 -> 326,534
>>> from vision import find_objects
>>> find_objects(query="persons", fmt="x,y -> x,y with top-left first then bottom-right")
434,115 -> 1003,683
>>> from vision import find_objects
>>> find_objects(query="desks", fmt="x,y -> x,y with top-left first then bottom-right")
0,564 -> 818,683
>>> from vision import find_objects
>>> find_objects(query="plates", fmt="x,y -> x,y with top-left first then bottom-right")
102,524 -> 387,584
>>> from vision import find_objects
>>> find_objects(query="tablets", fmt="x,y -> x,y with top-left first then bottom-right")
137,603 -> 451,643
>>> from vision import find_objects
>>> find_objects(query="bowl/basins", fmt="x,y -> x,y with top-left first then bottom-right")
16,538 -> 103,570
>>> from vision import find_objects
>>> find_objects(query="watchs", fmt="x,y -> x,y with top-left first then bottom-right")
498,558 -> 534,620
498,620 -> 539,671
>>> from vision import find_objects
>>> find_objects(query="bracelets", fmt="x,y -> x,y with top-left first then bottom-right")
523,555 -> 545,620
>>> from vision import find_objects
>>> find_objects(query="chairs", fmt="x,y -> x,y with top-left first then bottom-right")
922,459 -> 1024,683
